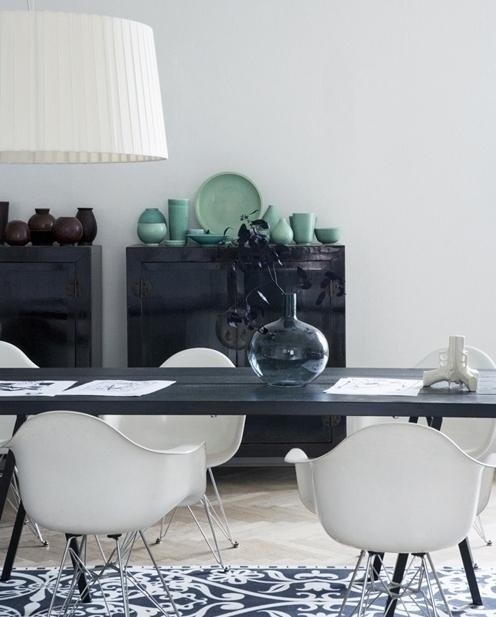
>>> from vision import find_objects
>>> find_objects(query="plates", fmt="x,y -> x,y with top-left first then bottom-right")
193,170 -> 264,241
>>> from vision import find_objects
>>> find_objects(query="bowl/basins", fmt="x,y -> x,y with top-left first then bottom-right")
137,206 -> 166,223
314,228 -> 342,243
136,223 -> 167,246
187,234 -> 226,246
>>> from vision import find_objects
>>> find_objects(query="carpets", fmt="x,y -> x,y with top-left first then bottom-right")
0,564 -> 496,617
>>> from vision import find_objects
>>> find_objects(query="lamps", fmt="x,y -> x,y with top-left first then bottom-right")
0,11 -> 169,167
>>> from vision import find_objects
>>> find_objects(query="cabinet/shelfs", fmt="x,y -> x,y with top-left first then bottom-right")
123,243 -> 347,468
0,244 -> 105,365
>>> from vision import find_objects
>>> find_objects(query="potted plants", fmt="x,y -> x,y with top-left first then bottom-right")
222,208 -> 346,387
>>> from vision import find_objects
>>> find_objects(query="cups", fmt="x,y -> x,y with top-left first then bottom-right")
293,214 -> 314,244
169,198 -> 189,240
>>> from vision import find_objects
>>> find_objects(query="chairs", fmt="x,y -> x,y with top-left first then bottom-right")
282,422 -> 496,617
94,346 -> 240,573
0,340 -> 51,551
0,410 -> 210,617
357,346 -> 496,590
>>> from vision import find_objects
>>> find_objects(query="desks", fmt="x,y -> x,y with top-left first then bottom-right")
1,367 -> 496,616
